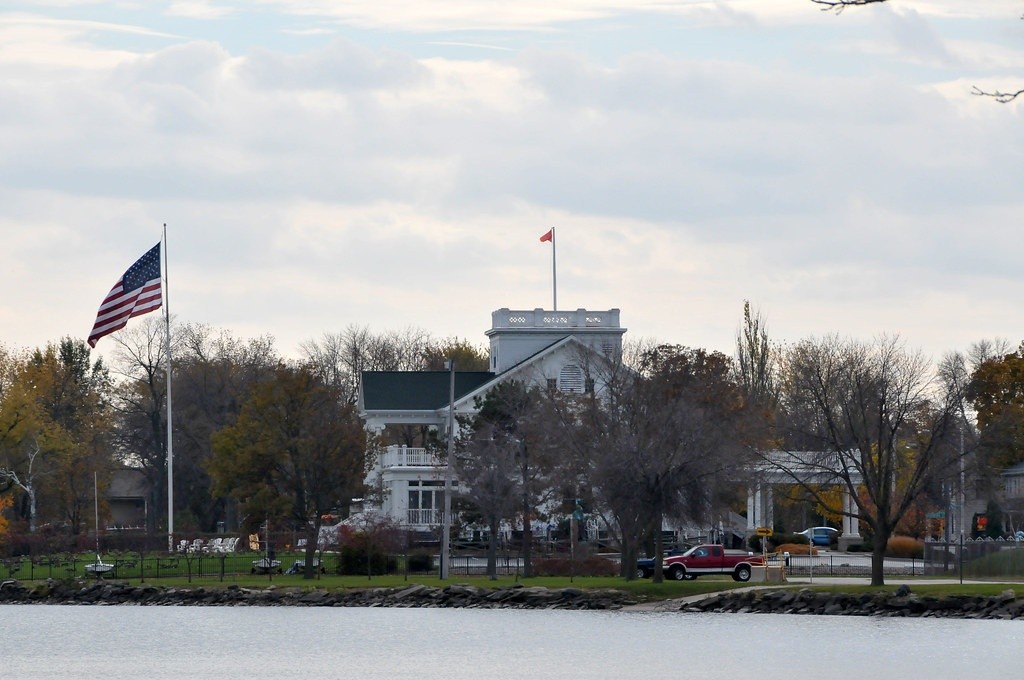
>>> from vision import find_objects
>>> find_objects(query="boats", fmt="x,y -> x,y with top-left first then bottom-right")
295,560 -> 325,574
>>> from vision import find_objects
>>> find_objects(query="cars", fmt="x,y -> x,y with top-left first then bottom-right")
793,527 -> 838,547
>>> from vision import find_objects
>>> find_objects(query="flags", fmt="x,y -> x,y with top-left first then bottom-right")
87,242 -> 163,349
540,229 -> 552,242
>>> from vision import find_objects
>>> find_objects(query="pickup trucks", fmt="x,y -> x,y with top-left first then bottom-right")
663,544 -> 765,581
636,551 -> 697,580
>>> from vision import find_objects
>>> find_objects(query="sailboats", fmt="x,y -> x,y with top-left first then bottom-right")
85,472 -> 115,575
252,514 -> 281,575
